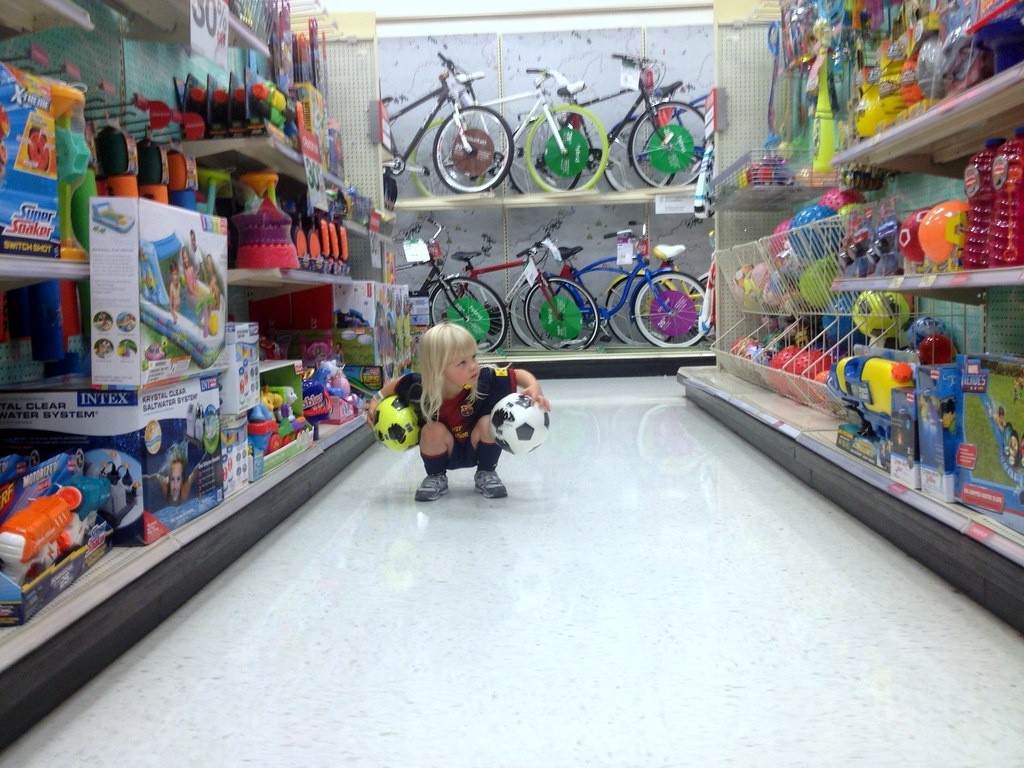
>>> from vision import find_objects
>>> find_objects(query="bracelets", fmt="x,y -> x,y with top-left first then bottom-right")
373,390 -> 384,402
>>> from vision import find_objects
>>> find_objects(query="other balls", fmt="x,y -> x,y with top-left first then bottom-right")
734,188 -> 972,382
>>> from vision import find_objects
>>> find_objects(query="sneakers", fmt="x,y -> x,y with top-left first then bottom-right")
474,470 -> 508,497
415,474 -> 448,500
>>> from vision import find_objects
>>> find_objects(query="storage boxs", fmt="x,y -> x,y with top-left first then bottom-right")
0,372 -> 226,546
251,279 -> 429,424
88,195 -> 226,392
0,62 -> 62,257
834,352 -> 1024,538
220,411 -> 249,500
0,453 -> 117,627
218,322 -> 260,416
249,360 -> 315,482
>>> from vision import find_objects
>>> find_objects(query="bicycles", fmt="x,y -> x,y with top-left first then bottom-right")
380,51 -> 514,194
603,81 -> 709,192
394,218 -> 509,355
507,53 -> 706,195
450,232 -> 601,351
509,221 -> 712,350
409,68 -> 610,198
605,230 -> 715,345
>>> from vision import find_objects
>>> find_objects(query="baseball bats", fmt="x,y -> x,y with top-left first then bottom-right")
810,47 -> 834,172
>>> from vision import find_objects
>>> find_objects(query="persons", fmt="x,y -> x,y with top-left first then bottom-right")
374,283 -> 412,378
182,245 -> 197,299
168,261 -> 182,324
333,343 -> 345,368
187,229 -> 208,284
139,440 -> 210,506
206,253 -> 220,310
365,322 -> 554,503
92,311 -> 137,359
988,374 -> 1024,470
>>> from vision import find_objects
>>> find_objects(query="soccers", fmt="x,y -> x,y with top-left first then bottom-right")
374,394 -> 425,452
851,290 -> 910,339
489,393 -> 550,455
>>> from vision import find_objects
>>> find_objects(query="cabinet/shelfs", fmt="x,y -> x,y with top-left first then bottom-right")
678,0 -> 1024,634
389,183 -> 711,380
0,0 -> 378,751
